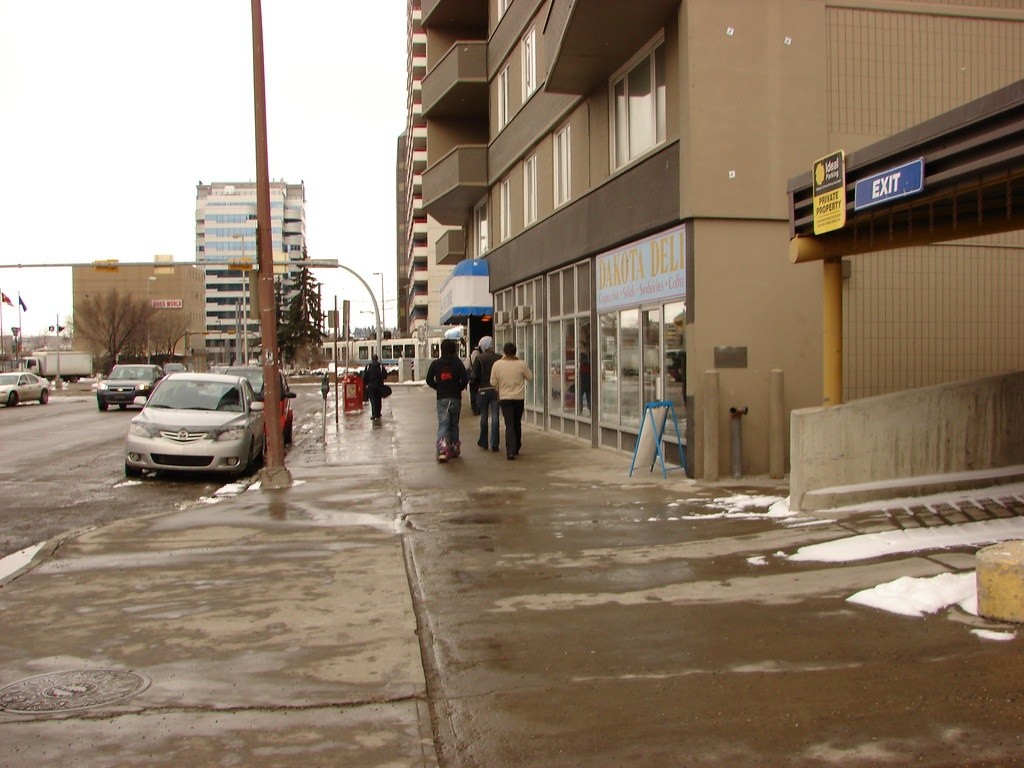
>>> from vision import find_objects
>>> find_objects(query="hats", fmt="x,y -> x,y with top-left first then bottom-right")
479,337 -> 492,350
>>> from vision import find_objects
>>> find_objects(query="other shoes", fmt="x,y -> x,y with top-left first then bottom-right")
492,447 -> 498,451
478,441 -> 488,449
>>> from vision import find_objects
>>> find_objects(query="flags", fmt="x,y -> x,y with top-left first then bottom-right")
19,296 -> 27,312
1,292 -> 14,307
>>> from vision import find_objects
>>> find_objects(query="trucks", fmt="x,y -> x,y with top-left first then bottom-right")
615,344 -> 660,376
19,351 -> 93,383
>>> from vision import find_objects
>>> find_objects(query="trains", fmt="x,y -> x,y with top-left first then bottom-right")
320,337 -> 447,366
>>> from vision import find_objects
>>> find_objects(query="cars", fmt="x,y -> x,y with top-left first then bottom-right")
565,359 -> 575,370
0,372 -> 51,406
125,370 -> 266,479
223,366 -> 294,447
163,363 -> 185,373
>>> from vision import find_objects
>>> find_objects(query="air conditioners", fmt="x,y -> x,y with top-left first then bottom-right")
512,305 -> 536,323
493,310 -> 510,325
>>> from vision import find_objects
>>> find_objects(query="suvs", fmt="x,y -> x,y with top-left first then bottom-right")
98,363 -> 166,412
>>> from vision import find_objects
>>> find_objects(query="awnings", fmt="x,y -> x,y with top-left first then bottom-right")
440,259 -> 494,326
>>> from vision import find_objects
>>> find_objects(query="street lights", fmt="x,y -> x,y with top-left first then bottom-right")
148,277 -> 156,364
373,272 -> 384,336
216,320 -> 222,366
233,233 -> 248,366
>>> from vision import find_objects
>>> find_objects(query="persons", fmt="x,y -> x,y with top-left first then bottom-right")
463,336 -> 503,452
579,353 -> 591,418
490,342 -> 532,460
426,339 -> 468,464
364,354 -> 388,420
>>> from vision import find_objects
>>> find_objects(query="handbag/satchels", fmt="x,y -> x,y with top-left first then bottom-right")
379,385 -> 391,398
